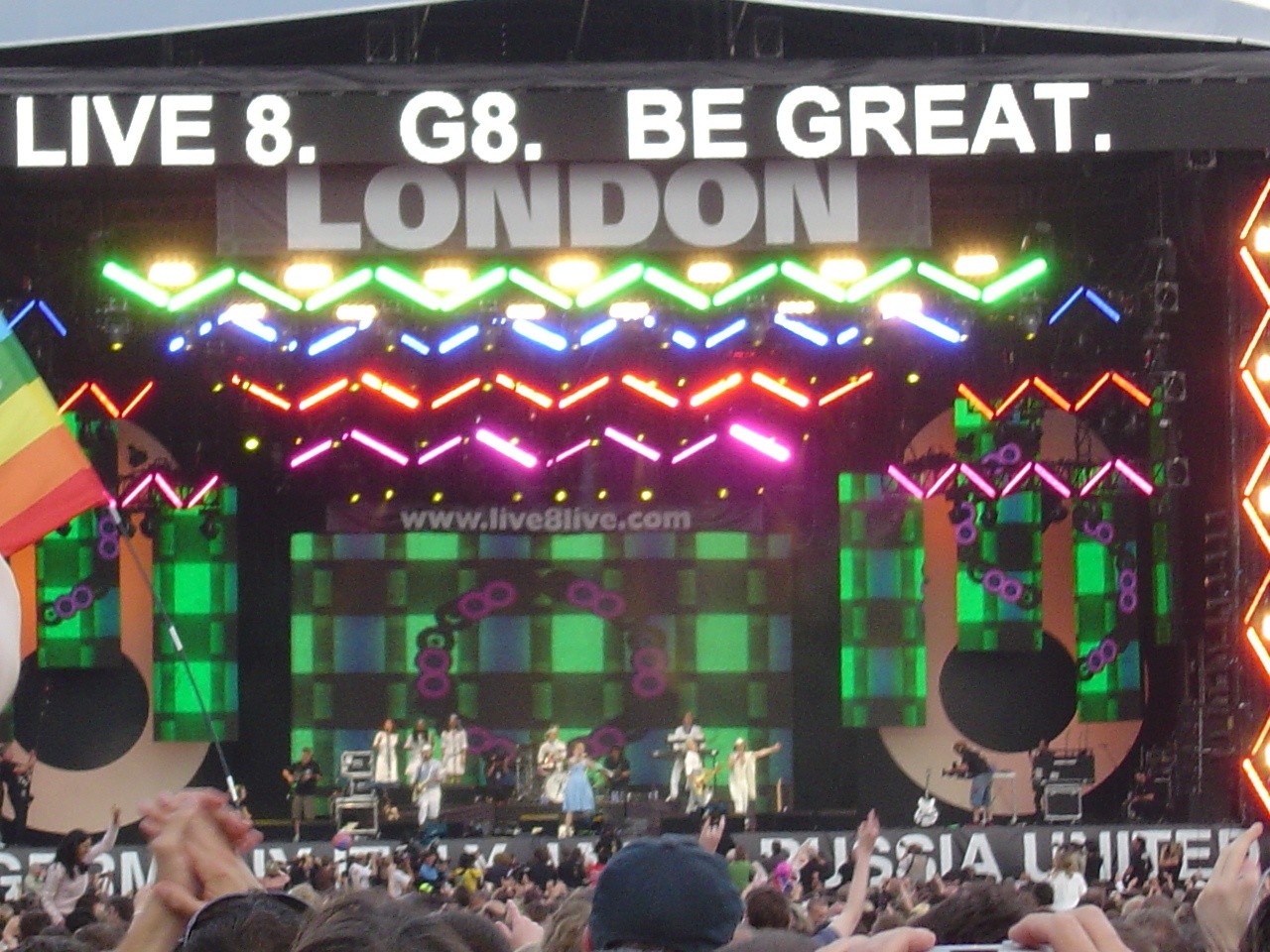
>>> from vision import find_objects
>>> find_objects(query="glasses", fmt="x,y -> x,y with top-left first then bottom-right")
183,893 -> 314,945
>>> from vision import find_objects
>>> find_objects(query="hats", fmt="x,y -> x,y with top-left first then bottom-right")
588,835 -> 744,951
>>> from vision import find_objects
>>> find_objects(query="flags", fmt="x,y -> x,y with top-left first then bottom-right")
0,309 -> 119,582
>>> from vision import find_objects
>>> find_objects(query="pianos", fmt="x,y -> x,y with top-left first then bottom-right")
649,731 -> 720,798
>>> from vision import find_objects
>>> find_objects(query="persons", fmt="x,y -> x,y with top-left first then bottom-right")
0,727 -> 1270,952
371,717 -> 400,823
663,712 -> 706,804
439,711 -> 470,785
534,722 -> 569,807
402,717 -> 436,786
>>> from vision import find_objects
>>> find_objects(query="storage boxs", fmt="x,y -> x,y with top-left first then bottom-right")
1044,784 -> 1083,824
334,751 -> 378,835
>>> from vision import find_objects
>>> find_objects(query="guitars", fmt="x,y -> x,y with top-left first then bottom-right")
912,769 -> 939,829
283,768 -> 326,786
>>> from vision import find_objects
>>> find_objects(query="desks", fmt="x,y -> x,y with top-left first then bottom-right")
982,770 -> 1017,826
